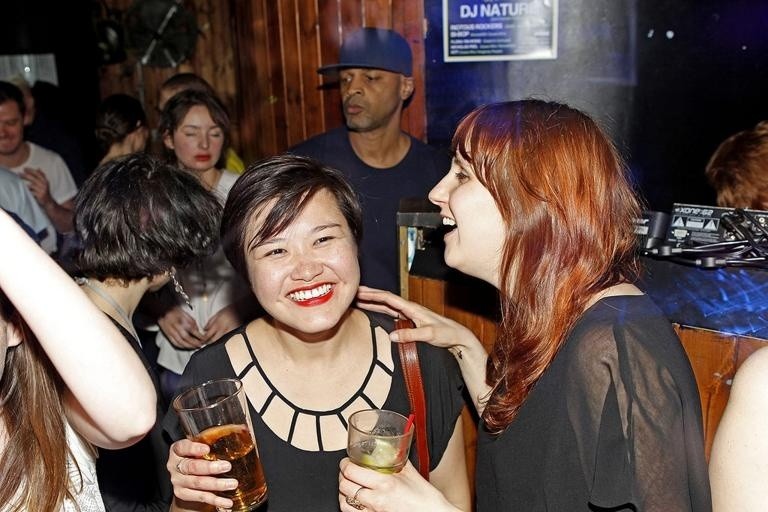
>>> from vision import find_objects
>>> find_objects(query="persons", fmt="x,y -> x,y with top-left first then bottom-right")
709,344 -> 768,512
160,155 -> 473,510
708,123 -> 767,210
288,26 -> 447,296
0,202 -> 157,510
337,96 -> 707,511
67,152 -> 227,511
2,72 -> 242,409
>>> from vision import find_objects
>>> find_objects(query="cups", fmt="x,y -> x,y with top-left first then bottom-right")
347,410 -> 414,473
173,378 -> 269,512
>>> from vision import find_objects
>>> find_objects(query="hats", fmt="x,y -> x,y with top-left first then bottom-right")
316,25 -> 413,79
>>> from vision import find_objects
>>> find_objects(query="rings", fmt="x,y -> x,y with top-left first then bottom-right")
397,313 -> 400,320
346,486 -> 366,511
